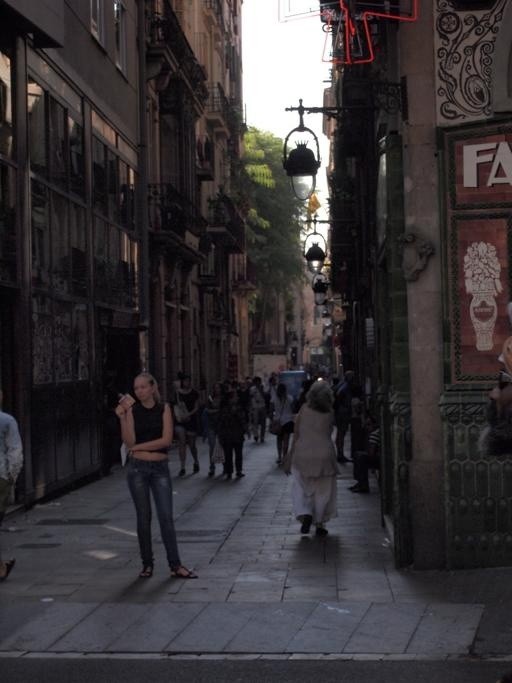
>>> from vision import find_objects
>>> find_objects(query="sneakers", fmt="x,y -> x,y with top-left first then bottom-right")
180,464 -> 199,475
208,466 -> 215,476
348,483 -> 369,493
227,472 -> 245,478
301,515 -> 328,536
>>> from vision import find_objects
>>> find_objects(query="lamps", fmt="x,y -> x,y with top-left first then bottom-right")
303,217 -> 357,275
311,273 -> 331,304
281,100 -> 379,201
314,299 -> 336,317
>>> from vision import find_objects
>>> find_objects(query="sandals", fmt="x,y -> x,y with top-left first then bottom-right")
139,565 -> 153,577
171,565 -> 197,579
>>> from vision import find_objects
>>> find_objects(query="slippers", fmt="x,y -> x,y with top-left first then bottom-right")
2,556 -> 16,580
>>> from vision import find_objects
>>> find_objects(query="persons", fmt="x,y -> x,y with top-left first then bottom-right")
0,390 -> 24,580
478,335 -> 512,455
116,373 -> 198,578
171,364 -> 381,492
286,381 -> 340,537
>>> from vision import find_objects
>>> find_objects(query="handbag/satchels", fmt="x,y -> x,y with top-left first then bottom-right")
268,420 -> 282,435
173,401 -> 190,423
